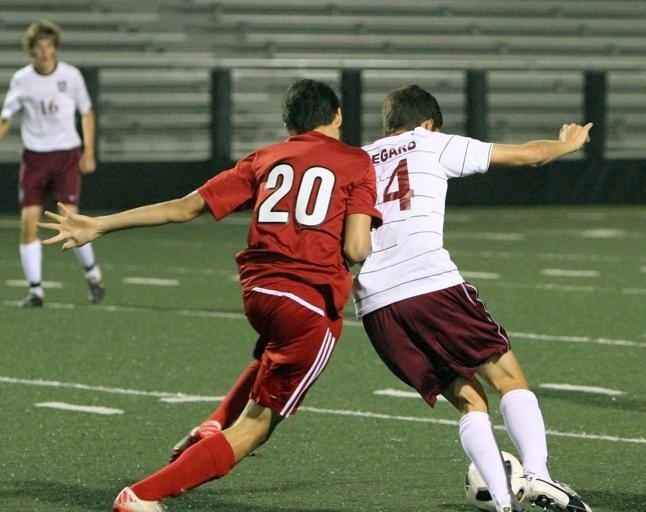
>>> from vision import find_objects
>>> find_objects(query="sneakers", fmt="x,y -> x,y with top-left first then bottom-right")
18,293 -> 44,307
113,487 -> 167,512
170,420 -> 221,462
85,274 -> 107,303
520,473 -> 590,511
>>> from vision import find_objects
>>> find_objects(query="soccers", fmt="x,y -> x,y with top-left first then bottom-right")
464,450 -> 527,512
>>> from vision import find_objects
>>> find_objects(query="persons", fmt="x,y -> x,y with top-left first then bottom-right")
0,18 -> 105,312
32,78 -> 378,512
341,83 -> 603,512
